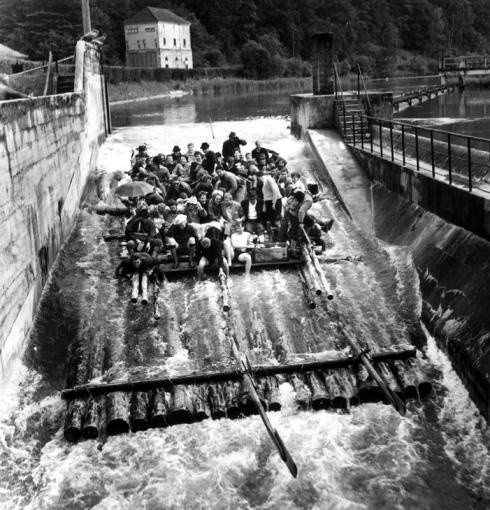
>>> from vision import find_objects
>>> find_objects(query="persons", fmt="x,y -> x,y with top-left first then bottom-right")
124,131 -> 319,278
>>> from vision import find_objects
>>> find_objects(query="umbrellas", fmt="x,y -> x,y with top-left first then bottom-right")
114,180 -> 155,201
